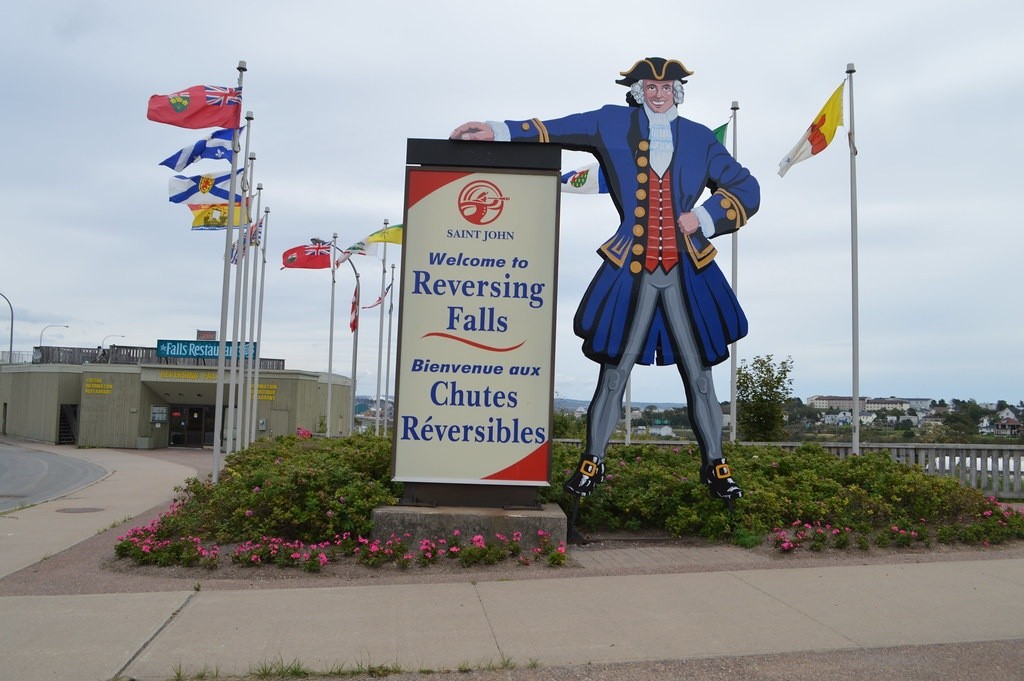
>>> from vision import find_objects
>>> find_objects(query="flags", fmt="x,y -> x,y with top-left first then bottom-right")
335,234 -> 379,269
369,223 -> 403,243
230,218 -> 262,264
362,283 -> 392,308
350,285 -> 359,333
280,242 -> 332,271
711,123 -> 728,146
158,126 -> 246,172
778,80 -> 842,178
147,85 -> 242,129
187,193 -> 257,231
168,166 -> 244,205
560,161 -> 610,195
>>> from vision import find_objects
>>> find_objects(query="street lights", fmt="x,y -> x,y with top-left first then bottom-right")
102,335 -> 125,350
39,324 -> 69,347
311,237 -> 360,437
